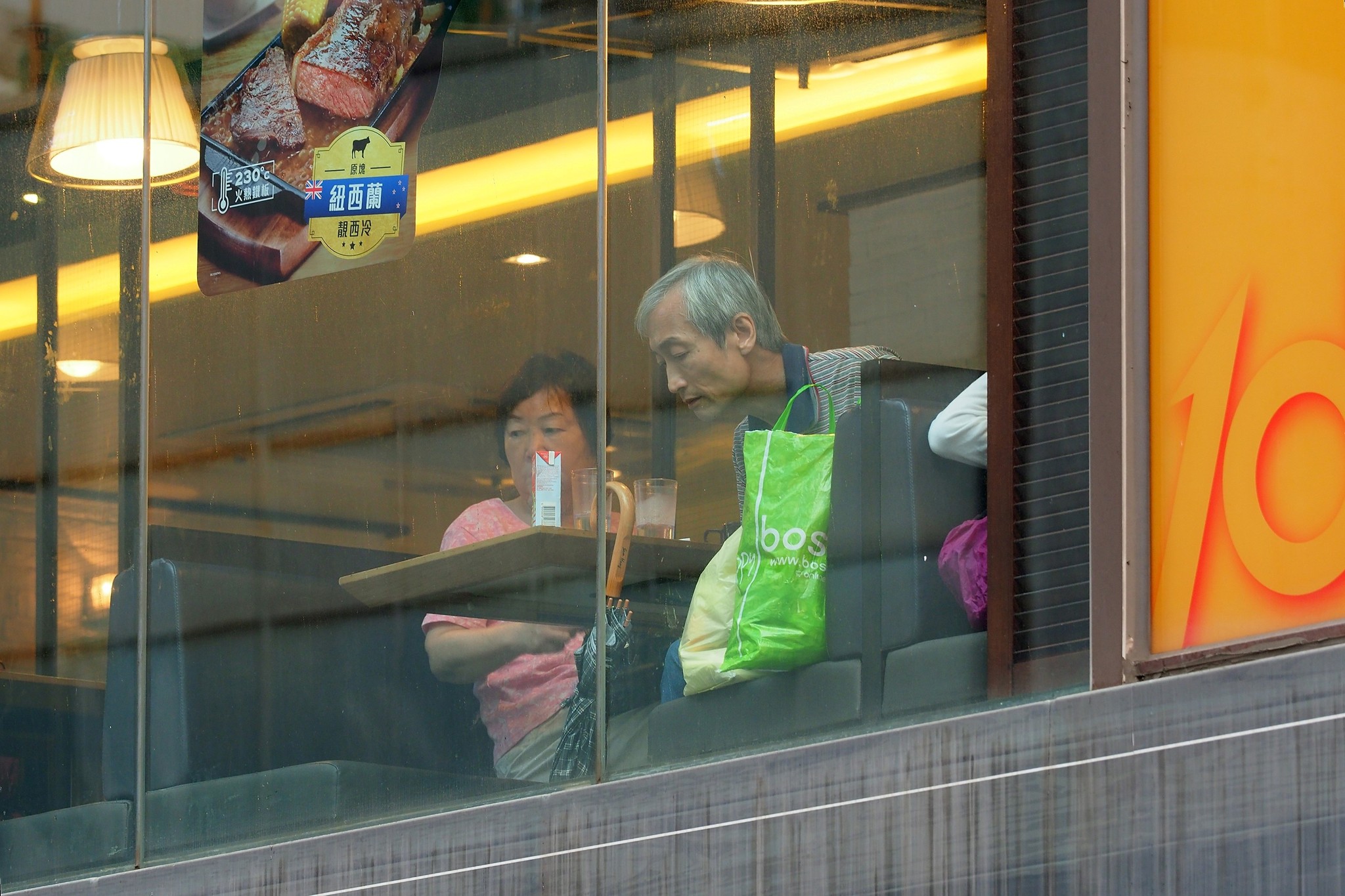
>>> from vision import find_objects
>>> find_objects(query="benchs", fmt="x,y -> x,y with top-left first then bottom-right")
644,407 -> 873,761
1,564 -> 136,884
877,394 -> 996,715
145,557 -> 562,870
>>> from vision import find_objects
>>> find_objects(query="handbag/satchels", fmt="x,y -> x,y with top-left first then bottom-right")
714,383 -> 837,675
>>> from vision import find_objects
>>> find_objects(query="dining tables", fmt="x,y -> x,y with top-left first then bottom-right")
338,523 -> 728,710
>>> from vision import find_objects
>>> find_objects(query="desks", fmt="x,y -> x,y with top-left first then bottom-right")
1,670 -> 107,828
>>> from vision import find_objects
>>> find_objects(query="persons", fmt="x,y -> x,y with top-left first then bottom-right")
632,252 -> 904,704
418,349 -> 659,783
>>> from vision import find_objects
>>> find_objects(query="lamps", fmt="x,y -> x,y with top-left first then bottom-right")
672,164 -> 725,251
25,2 -> 201,194
54,312 -> 119,385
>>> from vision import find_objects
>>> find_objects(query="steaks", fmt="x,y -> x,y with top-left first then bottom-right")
231,0 -> 424,160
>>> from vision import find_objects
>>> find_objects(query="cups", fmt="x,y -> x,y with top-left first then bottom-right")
570,468 -> 614,534
634,477 -> 677,539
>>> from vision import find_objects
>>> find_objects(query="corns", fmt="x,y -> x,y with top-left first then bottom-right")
280,0 -> 329,51
